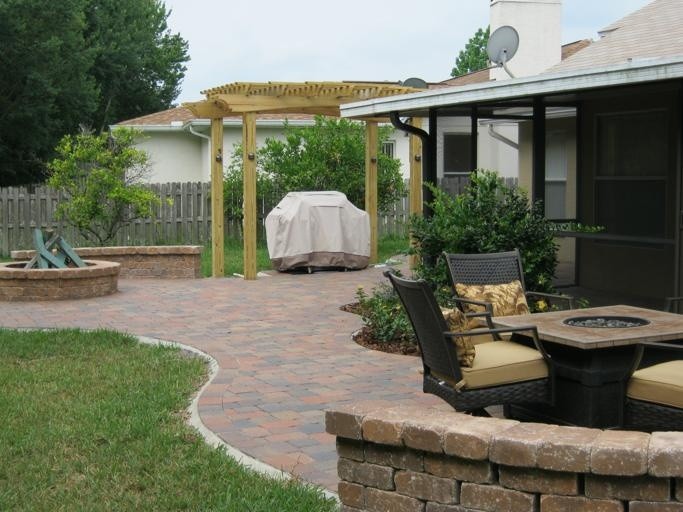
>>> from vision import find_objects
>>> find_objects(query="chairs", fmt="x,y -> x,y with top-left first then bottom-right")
381,247 -> 571,420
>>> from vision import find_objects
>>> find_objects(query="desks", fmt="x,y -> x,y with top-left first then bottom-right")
470,303 -> 683,430
